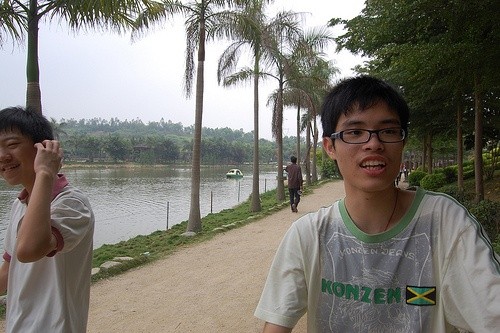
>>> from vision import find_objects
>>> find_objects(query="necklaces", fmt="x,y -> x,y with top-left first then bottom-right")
385,190 -> 398,231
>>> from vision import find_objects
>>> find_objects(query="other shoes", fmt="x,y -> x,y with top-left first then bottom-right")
292,204 -> 298,212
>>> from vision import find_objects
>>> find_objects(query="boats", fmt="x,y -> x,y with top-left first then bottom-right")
226,168 -> 244,179
275,165 -> 288,180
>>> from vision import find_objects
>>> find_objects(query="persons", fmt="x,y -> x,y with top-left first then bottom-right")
0,108 -> 95,333
286,156 -> 303,212
400,163 -> 408,177
254,76 -> 500,333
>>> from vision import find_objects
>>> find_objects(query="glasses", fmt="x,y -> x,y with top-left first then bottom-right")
330,127 -> 408,144
